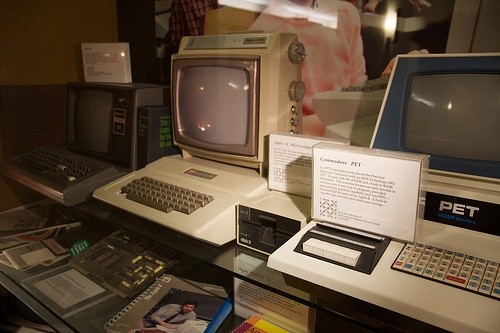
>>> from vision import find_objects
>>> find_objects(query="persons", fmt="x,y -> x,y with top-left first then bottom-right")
150,299 -> 200,329
243,0 -> 428,138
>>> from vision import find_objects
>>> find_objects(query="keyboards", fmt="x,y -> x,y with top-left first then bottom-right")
121,176 -> 214,215
341,77 -> 389,92
390,242 -> 500,301
20,148 -> 93,182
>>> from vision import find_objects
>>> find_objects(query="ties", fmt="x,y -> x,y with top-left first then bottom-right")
164,311 -> 182,322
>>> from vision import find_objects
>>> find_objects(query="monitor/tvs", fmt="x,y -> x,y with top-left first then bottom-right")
446,0 -> 500,53
370,53 -> 500,243
66,81 -> 170,173
170,32 -> 306,175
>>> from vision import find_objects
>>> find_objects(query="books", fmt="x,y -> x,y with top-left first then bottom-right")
231,315 -> 290,333
0,202 -> 234,333
